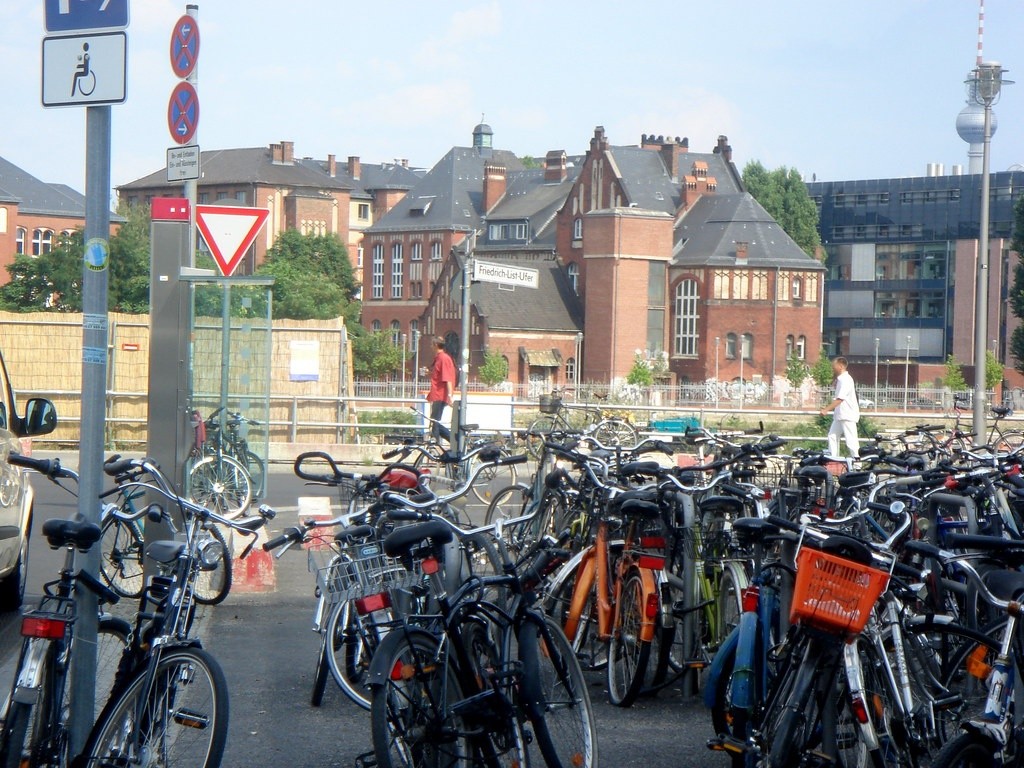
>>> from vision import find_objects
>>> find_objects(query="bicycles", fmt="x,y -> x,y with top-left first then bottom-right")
186,407 -> 266,520
0,451 -> 274,768
263,387 -> 1024,768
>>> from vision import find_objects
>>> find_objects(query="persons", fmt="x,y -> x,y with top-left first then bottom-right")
424,337 -> 456,448
820,357 -> 860,459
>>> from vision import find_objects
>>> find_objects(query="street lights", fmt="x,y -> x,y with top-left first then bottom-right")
904,336 -> 911,412
715,337 -> 720,409
574,332 -> 583,404
739,334 -> 745,409
414,331 -> 420,410
874,338 -> 880,413
402,334 -> 406,410
963,62 -> 1015,449
992,340 -> 996,392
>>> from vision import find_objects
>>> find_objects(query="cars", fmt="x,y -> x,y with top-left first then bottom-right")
0,351 -> 57,610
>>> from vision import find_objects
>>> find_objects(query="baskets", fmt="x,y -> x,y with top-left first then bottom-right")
539,394 -> 562,414
683,519 -> 747,561
789,547 -> 891,644
307,541 -> 431,604
749,464 -> 798,488
